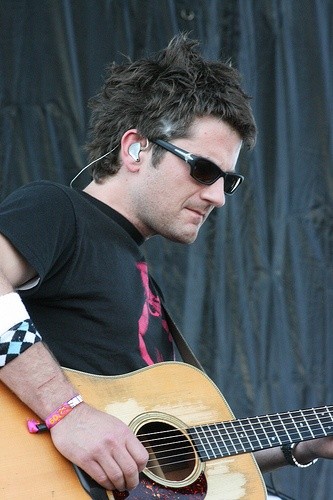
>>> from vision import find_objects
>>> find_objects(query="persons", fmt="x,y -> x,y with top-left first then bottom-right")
0,30 -> 333,498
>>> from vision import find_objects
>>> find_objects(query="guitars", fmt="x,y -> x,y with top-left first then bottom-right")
0,360 -> 332,500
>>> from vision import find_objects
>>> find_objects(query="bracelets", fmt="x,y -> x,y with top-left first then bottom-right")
279,446 -> 297,468
26,393 -> 84,433
290,443 -> 319,468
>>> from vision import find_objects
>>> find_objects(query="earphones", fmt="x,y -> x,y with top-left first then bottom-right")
128,142 -> 141,162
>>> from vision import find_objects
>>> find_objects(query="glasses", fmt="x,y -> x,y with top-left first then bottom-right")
148,137 -> 244,197
